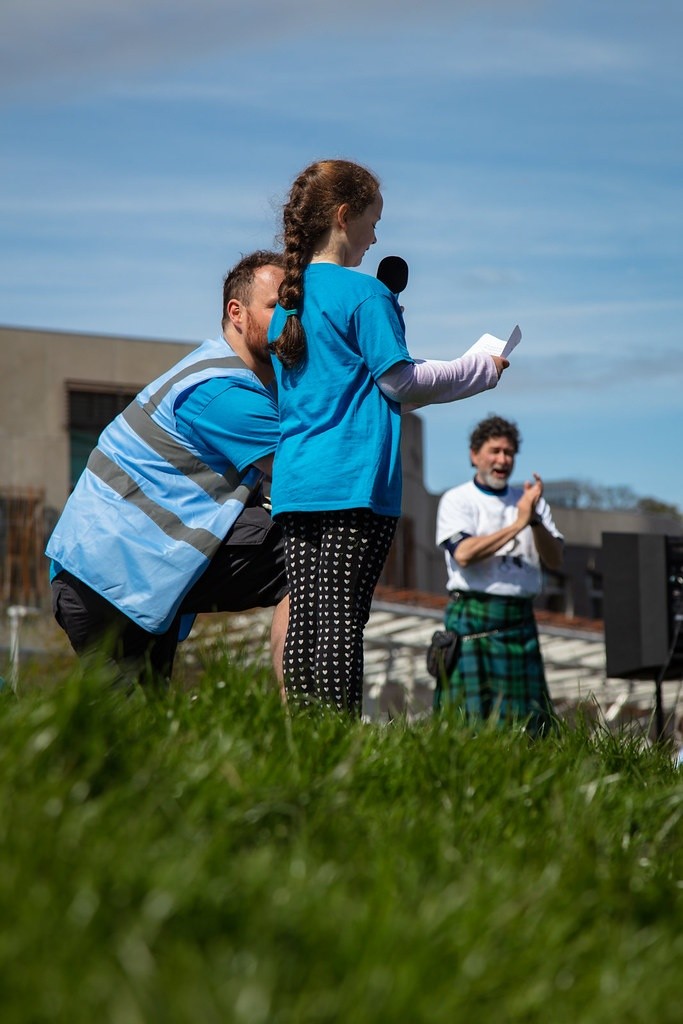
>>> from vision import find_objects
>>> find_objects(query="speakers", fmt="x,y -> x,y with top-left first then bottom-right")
602,530 -> 683,682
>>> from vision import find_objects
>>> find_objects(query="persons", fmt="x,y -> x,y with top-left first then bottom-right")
433,417 -> 566,736
266,157 -> 511,730
39,250 -> 297,704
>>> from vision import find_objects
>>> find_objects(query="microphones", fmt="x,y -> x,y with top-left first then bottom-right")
376,256 -> 408,300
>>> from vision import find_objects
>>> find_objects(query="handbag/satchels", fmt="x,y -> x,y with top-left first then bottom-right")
426,630 -> 462,681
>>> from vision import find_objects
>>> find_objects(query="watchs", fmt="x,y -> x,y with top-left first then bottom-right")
529,513 -> 542,526
